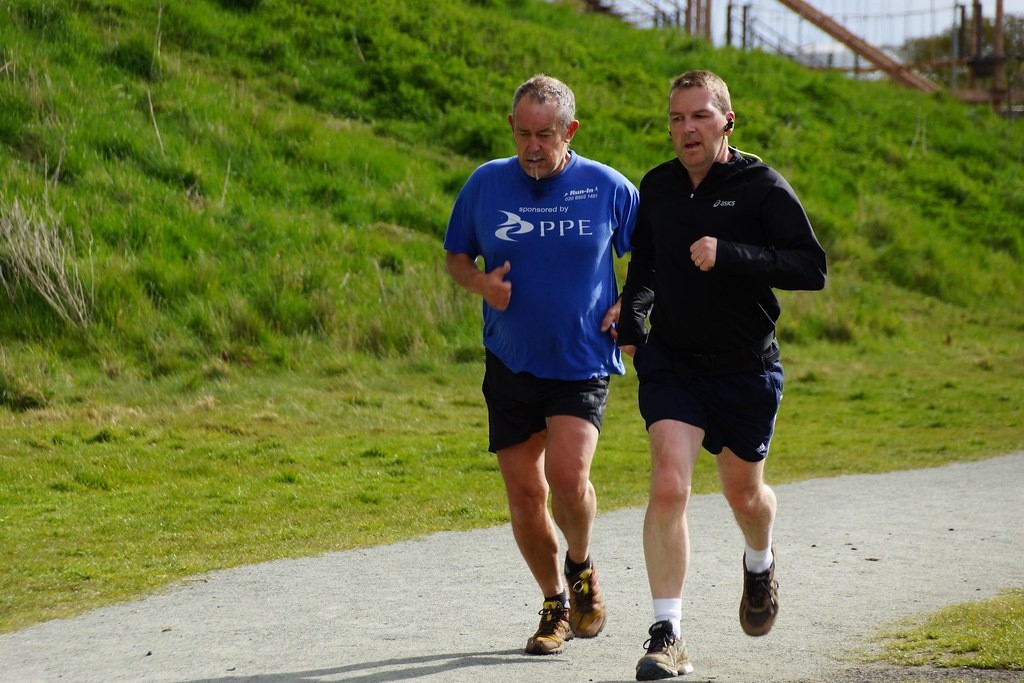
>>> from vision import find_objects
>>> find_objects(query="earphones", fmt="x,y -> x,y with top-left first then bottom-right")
724,118 -> 733,132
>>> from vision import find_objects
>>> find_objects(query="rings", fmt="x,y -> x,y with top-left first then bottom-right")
695,256 -> 702,264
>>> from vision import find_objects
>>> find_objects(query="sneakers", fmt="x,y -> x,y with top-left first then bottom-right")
636,618 -> 694,680
564,550 -> 607,638
526,601 -> 576,654
739,547 -> 779,637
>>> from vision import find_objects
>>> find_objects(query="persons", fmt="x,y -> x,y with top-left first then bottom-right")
616,69 -> 828,679
444,73 -> 640,656
